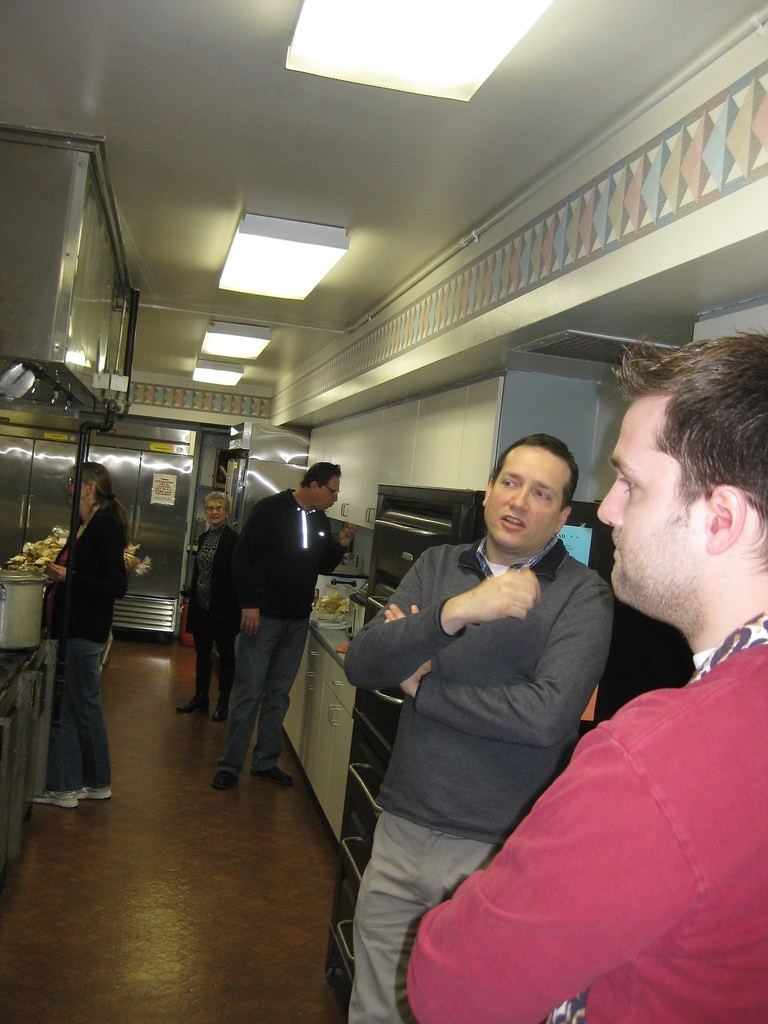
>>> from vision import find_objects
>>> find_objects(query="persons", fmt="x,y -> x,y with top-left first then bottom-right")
31,462 -> 130,809
211,463 -> 356,791
343,432 -> 615,1024
176,491 -> 243,721
407,335 -> 768,1024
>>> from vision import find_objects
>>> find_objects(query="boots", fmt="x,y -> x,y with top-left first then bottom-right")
211,695 -> 229,721
177,687 -> 209,713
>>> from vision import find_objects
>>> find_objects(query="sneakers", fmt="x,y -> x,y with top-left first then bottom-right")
78,784 -> 113,800
34,789 -> 78,808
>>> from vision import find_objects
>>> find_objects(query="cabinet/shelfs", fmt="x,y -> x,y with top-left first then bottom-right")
307,369 -> 629,531
283,631 -> 356,856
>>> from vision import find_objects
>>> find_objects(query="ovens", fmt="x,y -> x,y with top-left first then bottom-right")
324,484 -> 490,1002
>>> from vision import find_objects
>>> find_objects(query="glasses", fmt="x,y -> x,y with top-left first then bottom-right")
206,505 -> 226,512
316,479 -> 340,498
68,476 -> 91,487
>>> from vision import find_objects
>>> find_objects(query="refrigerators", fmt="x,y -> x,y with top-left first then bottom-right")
226,422 -> 311,537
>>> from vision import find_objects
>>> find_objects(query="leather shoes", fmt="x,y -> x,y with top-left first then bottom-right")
211,770 -> 239,789
250,767 -> 293,786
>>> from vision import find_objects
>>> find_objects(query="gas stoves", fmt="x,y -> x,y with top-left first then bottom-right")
0,644 -> 37,695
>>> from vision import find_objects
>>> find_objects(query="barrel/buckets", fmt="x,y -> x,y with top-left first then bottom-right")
0,568 -> 47,651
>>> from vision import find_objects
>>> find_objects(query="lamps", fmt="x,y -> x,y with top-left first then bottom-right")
284,0 -> 557,103
192,360 -> 244,387
200,321 -> 271,359
219,213 -> 349,300
